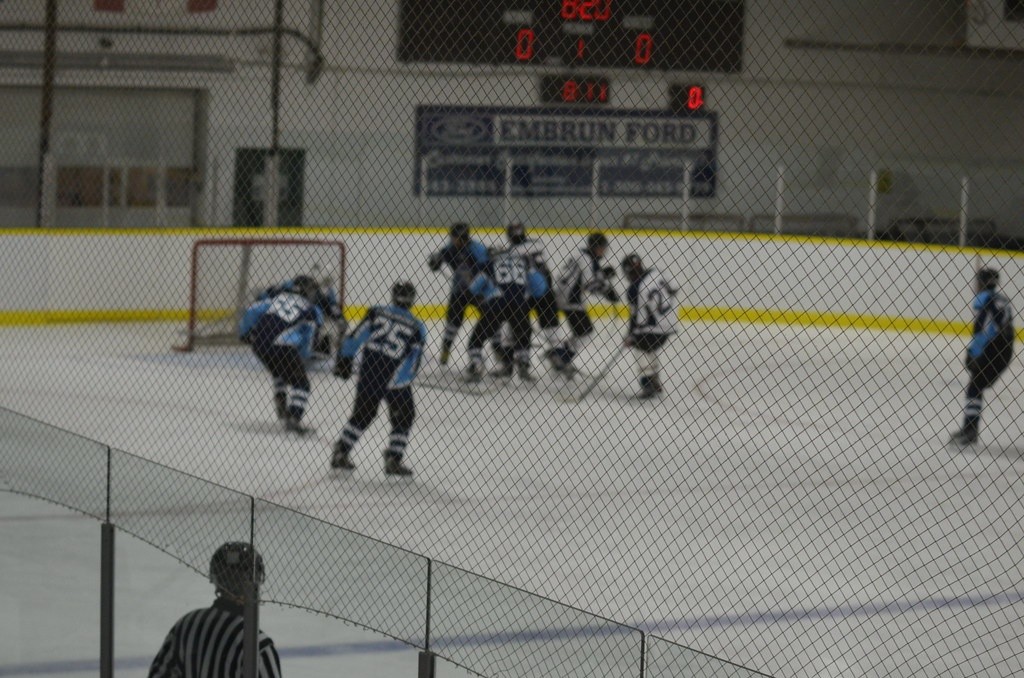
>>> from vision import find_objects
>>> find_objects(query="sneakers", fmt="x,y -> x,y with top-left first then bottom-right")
385,457 -> 412,479
440,355 -> 582,384
330,456 -> 355,478
950,428 -> 978,449
640,385 -> 661,399
286,422 -> 317,437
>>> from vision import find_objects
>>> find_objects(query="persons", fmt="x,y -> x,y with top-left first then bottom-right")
330,283 -> 426,476
148,542 -> 283,678
429,220 -> 489,366
459,221 -> 620,383
622,255 -> 678,399
239,273 -> 347,436
950,267 -> 1014,446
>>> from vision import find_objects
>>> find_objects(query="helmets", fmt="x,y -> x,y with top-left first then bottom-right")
509,224 -> 524,240
392,281 -> 415,299
978,271 -> 998,287
450,223 -> 469,240
209,542 -> 265,590
623,256 -> 640,271
295,275 -> 319,291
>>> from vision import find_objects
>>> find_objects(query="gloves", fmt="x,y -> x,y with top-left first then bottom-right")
604,267 -> 614,279
965,356 -> 976,372
605,291 -> 619,303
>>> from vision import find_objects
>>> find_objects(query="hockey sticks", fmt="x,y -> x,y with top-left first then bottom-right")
351,368 -> 501,395
549,341 -> 625,403
